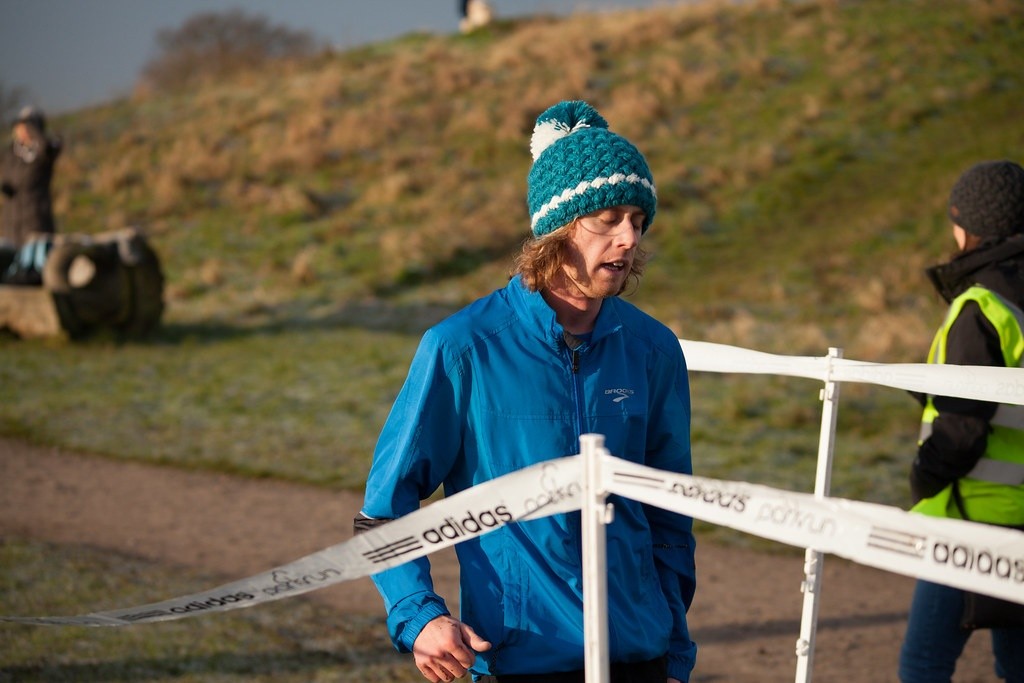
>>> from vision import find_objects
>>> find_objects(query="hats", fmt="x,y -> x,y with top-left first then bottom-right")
940,160 -> 1024,240
8,107 -> 45,128
524,98 -> 664,230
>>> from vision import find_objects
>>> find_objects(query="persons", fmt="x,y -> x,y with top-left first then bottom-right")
354,98 -> 698,683
897,160 -> 1024,683
0,103 -> 64,267
